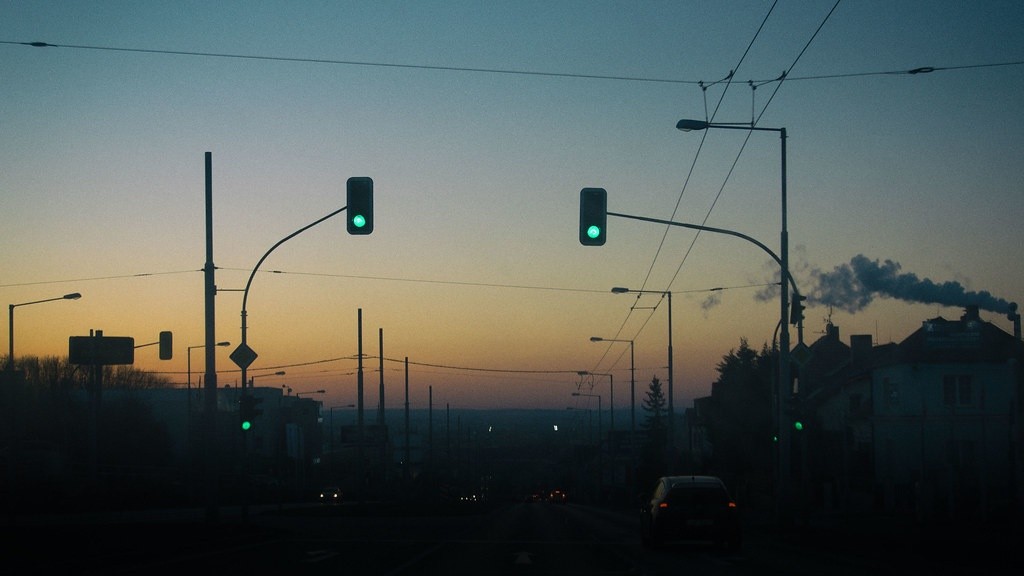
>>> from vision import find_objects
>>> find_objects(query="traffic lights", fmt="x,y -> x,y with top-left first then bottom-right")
580,188 -> 607,246
790,293 -> 807,324
240,397 -> 255,432
347,177 -> 373,235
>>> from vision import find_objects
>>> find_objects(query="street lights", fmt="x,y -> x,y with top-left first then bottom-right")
296,389 -> 326,463
252,371 -> 286,397
572,392 -> 602,464
9,293 -> 81,440
188,342 -> 230,449
590,337 -> 635,425
578,370 -> 615,485
331,404 -> 356,451
612,287 -> 674,463
675,119 -> 790,499
567,407 -> 592,444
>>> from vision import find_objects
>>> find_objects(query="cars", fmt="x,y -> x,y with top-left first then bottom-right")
639,475 -> 742,554
532,488 -> 569,505
